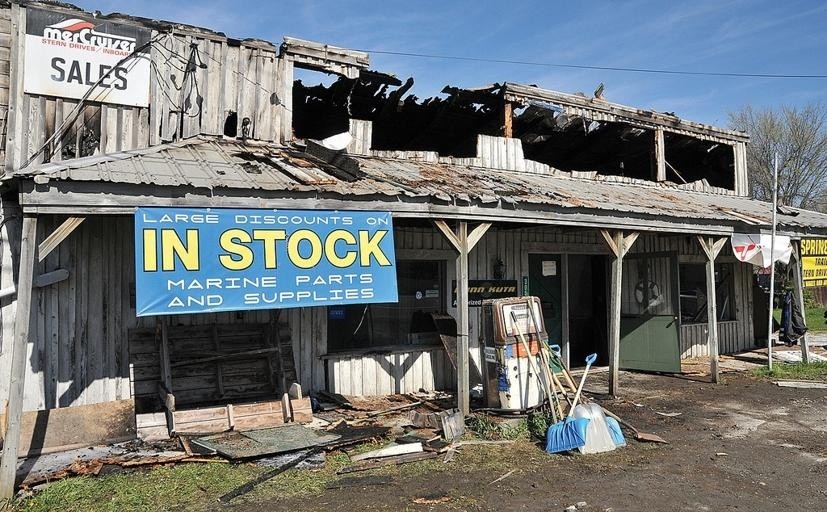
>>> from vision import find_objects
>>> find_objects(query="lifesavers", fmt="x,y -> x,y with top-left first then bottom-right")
637,281 -> 659,307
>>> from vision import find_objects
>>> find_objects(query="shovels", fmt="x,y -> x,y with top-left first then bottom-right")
510,296 -> 665,456
